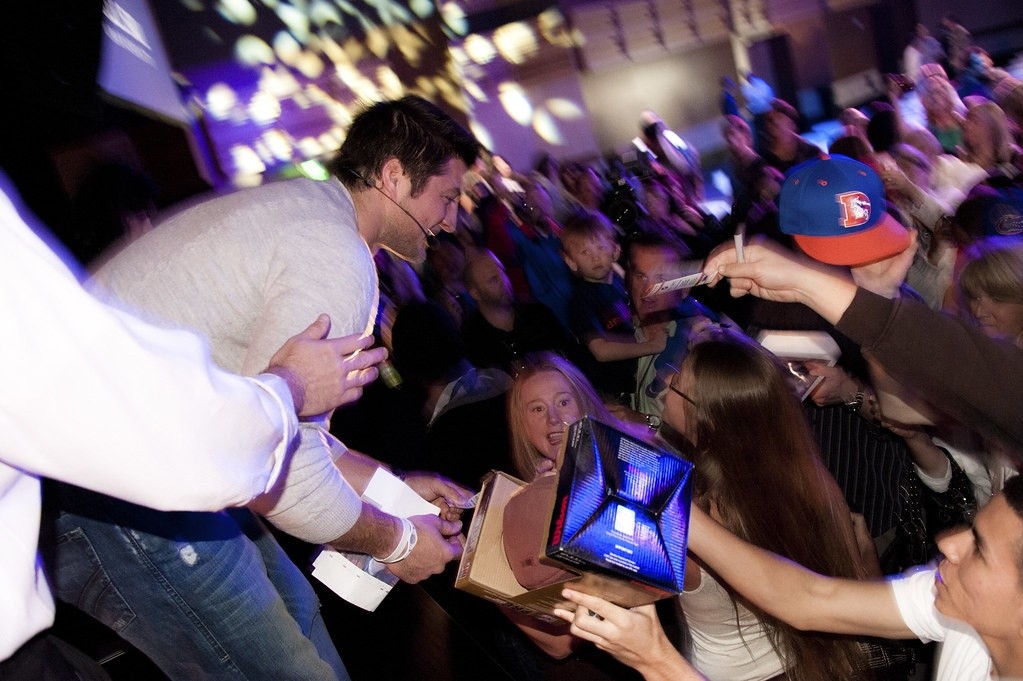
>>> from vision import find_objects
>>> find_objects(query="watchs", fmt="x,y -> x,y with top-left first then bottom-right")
644,414 -> 661,436
844,381 -> 864,414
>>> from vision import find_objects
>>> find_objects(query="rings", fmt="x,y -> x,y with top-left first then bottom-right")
892,180 -> 895,185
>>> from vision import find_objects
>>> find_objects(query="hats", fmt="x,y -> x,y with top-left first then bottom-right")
778,153 -> 911,266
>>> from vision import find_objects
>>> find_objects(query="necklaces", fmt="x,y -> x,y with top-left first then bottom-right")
632,356 -> 654,411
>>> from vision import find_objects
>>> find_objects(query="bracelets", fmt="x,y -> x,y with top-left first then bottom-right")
371,516 -> 418,564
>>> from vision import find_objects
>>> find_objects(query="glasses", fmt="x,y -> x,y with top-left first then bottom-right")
934,213 -> 952,234
669,369 -> 698,408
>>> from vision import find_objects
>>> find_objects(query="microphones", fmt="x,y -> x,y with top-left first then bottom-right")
344,163 -> 442,248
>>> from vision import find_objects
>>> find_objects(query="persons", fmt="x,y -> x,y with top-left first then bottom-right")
42,93 -> 480,681
0,186 -> 391,681
399,14 -> 1022,681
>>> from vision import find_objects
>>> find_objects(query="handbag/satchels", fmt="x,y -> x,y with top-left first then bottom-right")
879,446 -> 981,666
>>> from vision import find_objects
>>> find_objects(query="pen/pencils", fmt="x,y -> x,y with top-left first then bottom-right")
733,228 -> 746,262
